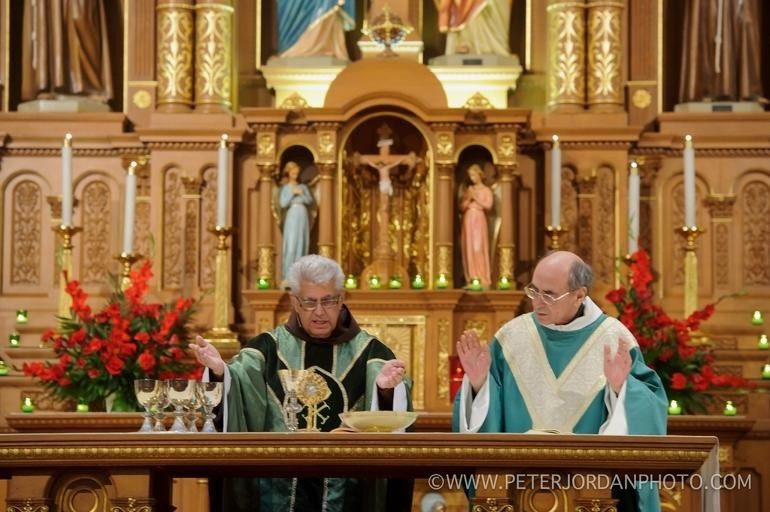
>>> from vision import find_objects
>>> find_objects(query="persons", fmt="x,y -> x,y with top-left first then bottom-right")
190,253 -> 417,512
448,246 -> 672,511
458,161 -> 496,290
353,156 -> 407,211
278,160 -> 315,281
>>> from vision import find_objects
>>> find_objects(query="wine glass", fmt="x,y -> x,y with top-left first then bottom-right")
130,373 -> 226,435
275,367 -> 308,433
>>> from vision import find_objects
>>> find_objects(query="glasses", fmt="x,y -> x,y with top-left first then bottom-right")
295,295 -> 342,309
524,282 -> 574,303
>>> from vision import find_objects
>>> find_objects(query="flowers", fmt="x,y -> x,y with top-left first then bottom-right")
12,258 -> 203,407
595,252 -> 756,412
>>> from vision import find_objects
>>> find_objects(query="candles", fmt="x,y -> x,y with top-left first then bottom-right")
0,307 -> 88,412
669,320 -> 770,417
257,275 -> 513,291
60,140 -> 229,254
550,141 -> 697,255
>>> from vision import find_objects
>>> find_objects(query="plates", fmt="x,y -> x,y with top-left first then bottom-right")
336,408 -> 421,436
